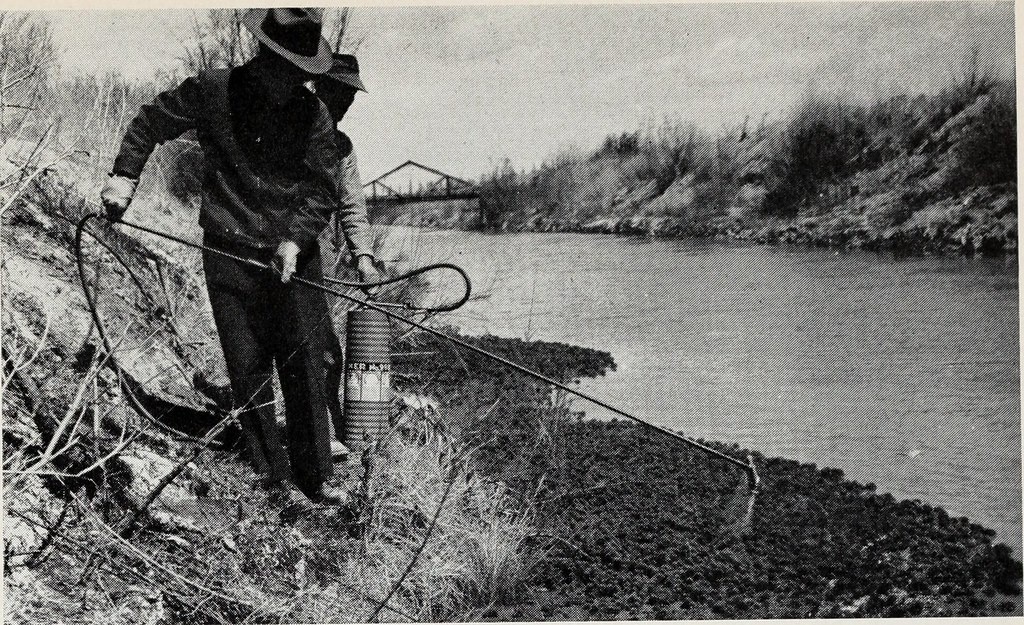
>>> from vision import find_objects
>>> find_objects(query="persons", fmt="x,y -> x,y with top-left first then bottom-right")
101,9 -> 379,512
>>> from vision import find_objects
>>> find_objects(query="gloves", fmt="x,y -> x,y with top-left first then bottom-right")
268,243 -> 301,284
103,174 -> 137,223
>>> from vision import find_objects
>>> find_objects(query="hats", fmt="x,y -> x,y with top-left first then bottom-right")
244,6 -> 334,75
320,53 -> 368,94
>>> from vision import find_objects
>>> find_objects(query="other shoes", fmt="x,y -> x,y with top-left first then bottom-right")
313,483 -> 347,505
